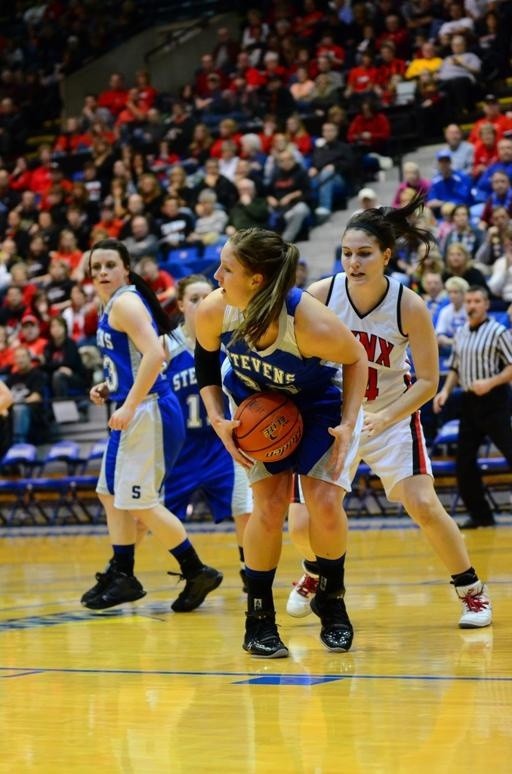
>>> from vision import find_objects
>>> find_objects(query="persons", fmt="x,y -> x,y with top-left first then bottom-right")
150,272 -> 258,576
74,239 -> 225,614
0,0 -> 512,447
282,186 -> 494,628
183,227 -> 369,659
427,287 -> 510,531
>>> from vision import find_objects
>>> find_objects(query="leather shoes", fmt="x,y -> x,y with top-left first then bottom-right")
457,518 -> 495,528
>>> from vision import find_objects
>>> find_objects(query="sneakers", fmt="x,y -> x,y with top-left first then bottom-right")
171,565 -> 223,612
242,559 -> 353,658
81,560 -> 147,609
455,580 -> 493,628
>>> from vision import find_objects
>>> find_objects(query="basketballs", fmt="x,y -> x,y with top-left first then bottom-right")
233,392 -> 305,462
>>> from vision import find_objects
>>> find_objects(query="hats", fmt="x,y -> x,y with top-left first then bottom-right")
21,315 -> 38,325
